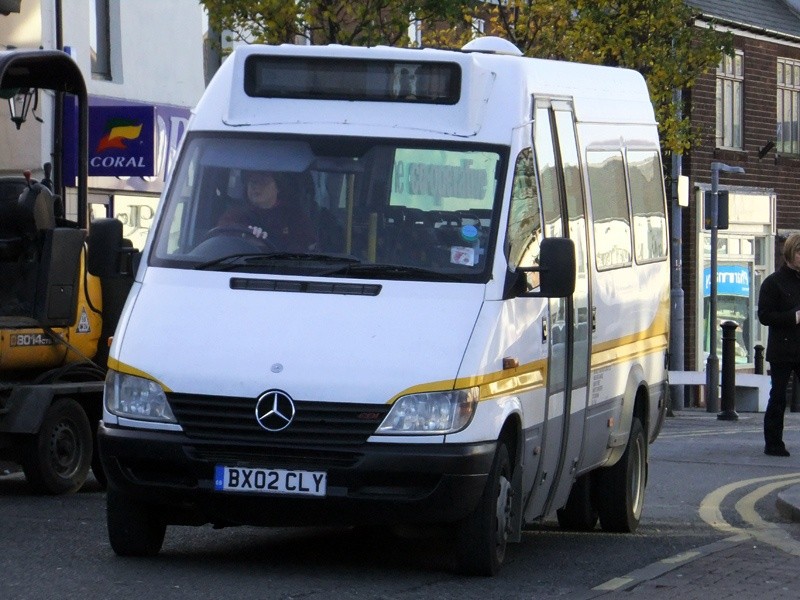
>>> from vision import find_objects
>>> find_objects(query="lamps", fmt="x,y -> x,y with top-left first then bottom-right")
758,140 -> 776,160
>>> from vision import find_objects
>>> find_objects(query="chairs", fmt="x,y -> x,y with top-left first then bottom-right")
358,201 -> 497,268
1,176 -> 39,279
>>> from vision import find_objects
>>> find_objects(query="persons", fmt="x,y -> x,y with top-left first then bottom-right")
214,171 -> 317,256
758,234 -> 800,456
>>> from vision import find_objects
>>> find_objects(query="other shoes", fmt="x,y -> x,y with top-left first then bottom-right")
764,442 -> 791,456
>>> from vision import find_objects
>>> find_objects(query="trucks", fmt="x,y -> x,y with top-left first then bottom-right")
109,35 -> 674,568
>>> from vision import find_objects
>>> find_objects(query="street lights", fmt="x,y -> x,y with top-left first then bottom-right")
705,160 -> 755,415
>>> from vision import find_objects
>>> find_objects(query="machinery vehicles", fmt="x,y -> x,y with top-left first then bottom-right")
1,45 -> 144,501
